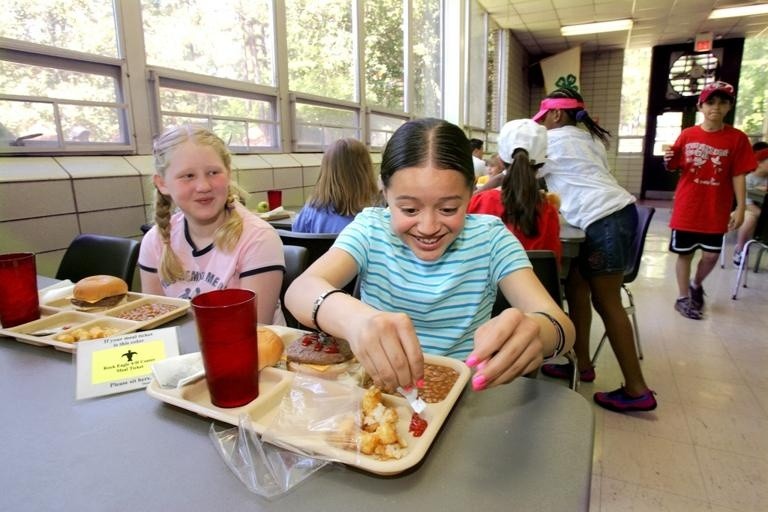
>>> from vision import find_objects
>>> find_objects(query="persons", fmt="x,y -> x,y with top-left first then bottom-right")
473,153 -> 500,194
469,139 -> 486,179
661,80 -> 760,320
731,141 -> 768,269
466,119 -> 563,273
136,122 -> 288,329
284,118 -> 577,394
474,87 -> 658,413
291,136 -> 385,234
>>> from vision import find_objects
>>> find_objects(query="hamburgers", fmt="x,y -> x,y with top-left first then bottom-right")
546,192 -> 560,210
66,274 -> 129,314
256,324 -> 357,378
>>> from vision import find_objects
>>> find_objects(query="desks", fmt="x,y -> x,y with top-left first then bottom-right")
1,268 -> 600,512
141,207 -> 591,261
746,190 -> 764,208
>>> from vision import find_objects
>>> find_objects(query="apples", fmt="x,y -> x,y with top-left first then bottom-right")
258,202 -> 269,213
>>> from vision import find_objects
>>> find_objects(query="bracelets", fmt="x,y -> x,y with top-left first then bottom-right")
312,290 -> 349,338
529,311 -> 565,363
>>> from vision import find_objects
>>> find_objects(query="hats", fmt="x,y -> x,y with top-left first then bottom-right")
700,82 -> 733,103
530,99 -> 584,121
499,119 -> 546,164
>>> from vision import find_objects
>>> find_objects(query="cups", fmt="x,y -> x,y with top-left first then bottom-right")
267,190 -> 282,211
192,288 -> 263,410
666,145 -> 681,170
1,251 -> 42,330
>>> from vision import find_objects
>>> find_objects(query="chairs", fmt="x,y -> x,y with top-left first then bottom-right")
729,194 -> 767,305
273,225 -> 361,337
490,247 -> 579,388
561,203 -> 654,371
53,230 -> 138,291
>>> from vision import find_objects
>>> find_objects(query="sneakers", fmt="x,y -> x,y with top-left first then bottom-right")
595,388 -> 656,410
540,361 -> 595,382
733,244 -> 742,269
675,297 -> 704,319
691,283 -> 704,309
